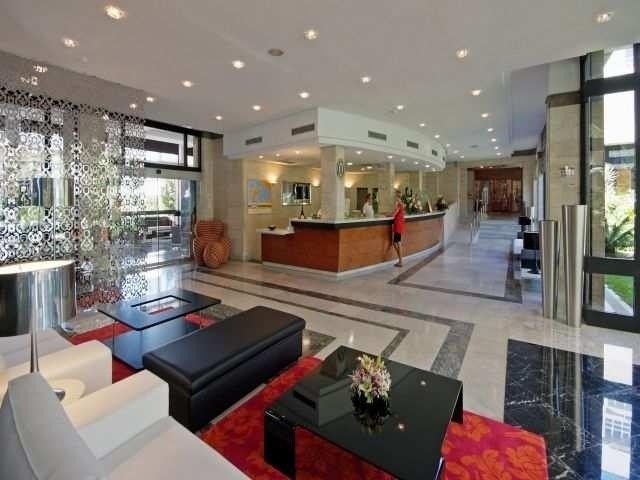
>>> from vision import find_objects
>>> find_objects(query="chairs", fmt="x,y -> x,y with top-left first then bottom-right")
2,328 -> 249,480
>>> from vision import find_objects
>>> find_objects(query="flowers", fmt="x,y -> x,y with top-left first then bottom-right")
347,351 -> 394,402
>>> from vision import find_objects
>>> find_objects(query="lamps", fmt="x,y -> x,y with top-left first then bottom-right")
1,260 -> 76,406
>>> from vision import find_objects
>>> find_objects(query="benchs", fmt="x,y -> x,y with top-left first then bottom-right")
142,306 -> 306,434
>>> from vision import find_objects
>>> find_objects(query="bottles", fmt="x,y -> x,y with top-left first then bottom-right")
300,207 -> 304,219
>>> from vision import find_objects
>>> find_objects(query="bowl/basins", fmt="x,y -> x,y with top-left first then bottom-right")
268,225 -> 276,230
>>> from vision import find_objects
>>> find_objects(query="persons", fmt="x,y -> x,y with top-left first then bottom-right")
362,193 -> 374,218
385,192 -> 405,268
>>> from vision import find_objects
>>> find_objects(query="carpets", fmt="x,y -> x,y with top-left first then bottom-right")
69,311 -> 548,478
